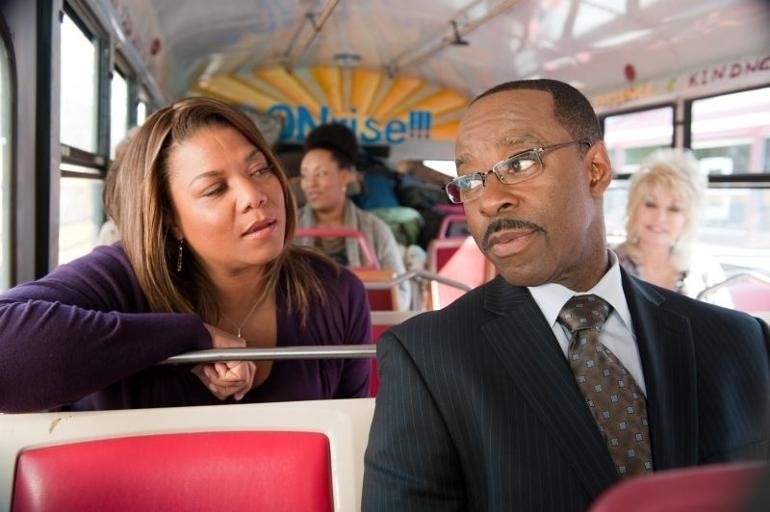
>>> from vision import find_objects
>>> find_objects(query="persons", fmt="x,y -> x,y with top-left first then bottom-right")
305,121 -> 402,215
286,141 -> 414,312
1,93 -> 376,418
353,75 -> 769,510
606,146 -> 737,313
88,122 -> 143,253
270,140 -> 308,210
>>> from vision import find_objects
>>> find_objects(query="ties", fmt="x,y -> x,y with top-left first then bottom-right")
556,297 -> 656,476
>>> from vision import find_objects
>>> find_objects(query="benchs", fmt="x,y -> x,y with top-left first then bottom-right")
0,201 -> 769,510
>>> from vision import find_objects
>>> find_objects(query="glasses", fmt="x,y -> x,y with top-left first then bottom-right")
445,140 -> 591,205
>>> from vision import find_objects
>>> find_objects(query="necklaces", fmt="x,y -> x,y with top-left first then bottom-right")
206,286 -> 270,340
